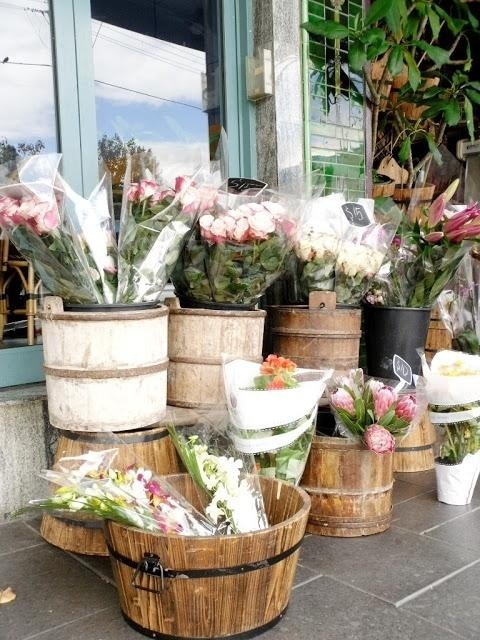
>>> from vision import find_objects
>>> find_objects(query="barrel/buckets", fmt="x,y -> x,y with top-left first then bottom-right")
33,288 -> 480,638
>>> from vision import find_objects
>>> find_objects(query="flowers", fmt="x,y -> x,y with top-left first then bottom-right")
0,439 -> 209,537
163,419 -> 268,534
169,185 -> 303,313
236,347 -> 315,484
371,185 -> 480,310
0,168 -> 119,312
420,345 -> 480,464
284,202 -> 394,308
113,166 -> 221,305
319,361 -> 419,454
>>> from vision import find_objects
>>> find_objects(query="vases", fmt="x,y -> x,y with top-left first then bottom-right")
265,284 -> 366,380
34,299 -> 175,438
167,294 -> 269,409
362,299 -> 430,383
89,470 -> 313,640
385,394 -> 439,472
299,409 -> 400,543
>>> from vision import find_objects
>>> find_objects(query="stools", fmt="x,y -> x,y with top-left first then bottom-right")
0,227 -> 50,348
